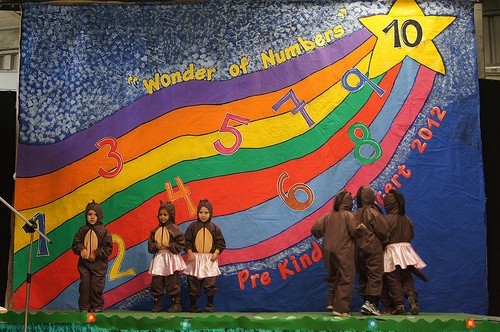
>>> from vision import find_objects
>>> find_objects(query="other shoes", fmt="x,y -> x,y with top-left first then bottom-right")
408,296 -> 419,315
205,303 -> 213,313
80,307 -> 91,313
93,308 -> 103,313
191,306 -> 202,313
391,305 -> 405,315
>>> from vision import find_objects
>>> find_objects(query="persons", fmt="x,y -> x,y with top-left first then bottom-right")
312,190 -> 366,317
184,199 -> 227,312
147,200 -> 184,312
72,200 -> 113,312
352,185 -> 387,313
383,189 -> 427,314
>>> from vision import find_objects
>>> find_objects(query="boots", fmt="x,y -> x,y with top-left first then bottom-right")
152,294 -> 162,312
167,297 -> 182,312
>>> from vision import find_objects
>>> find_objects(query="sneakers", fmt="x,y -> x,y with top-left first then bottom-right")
327,305 -> 353,317
361,301 -> 381,316
